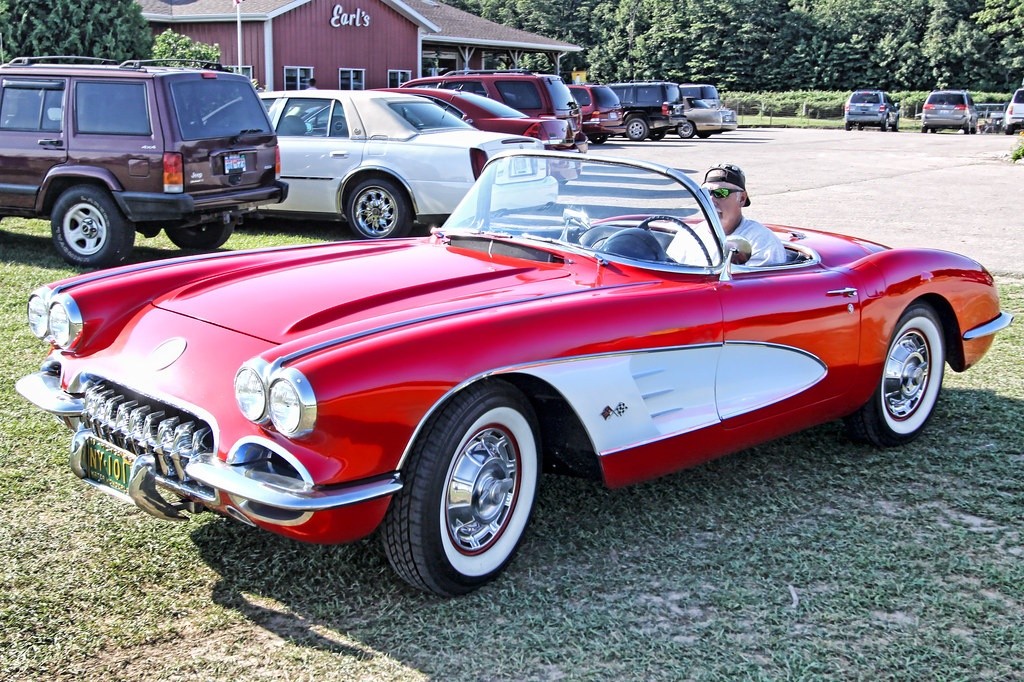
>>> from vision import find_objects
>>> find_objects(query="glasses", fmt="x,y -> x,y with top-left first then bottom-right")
700,188 -> 744,198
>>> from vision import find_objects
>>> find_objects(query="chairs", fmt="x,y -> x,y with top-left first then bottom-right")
329,114 -> 349,137
276,115 -> 308,136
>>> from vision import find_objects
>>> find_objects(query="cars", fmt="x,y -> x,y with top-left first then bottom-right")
14,152 -> 1013,598
372,88 -> 569,151
674,96 -> 739,138
192,91 -> 558,244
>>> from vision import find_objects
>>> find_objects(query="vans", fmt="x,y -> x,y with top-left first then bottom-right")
678,84 -> 721,108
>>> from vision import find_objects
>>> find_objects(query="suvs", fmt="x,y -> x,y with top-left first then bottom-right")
1003,88 -> 1024,134
606,81 -> 685,142
565,84 -> 627,144
844,90 -> 900,132
0,55 -> 288,268
396,69 -> 583,152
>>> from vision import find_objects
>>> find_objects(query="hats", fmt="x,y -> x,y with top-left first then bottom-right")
699,164 -> 750,207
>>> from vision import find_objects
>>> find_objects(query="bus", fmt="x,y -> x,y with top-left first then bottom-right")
922,91 -> 978,135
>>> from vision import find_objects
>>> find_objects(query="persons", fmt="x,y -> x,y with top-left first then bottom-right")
665,163 -> 786,267
302,78 -> 320,121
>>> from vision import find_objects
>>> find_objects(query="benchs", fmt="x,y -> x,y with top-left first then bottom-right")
575,225 -> 805,263
